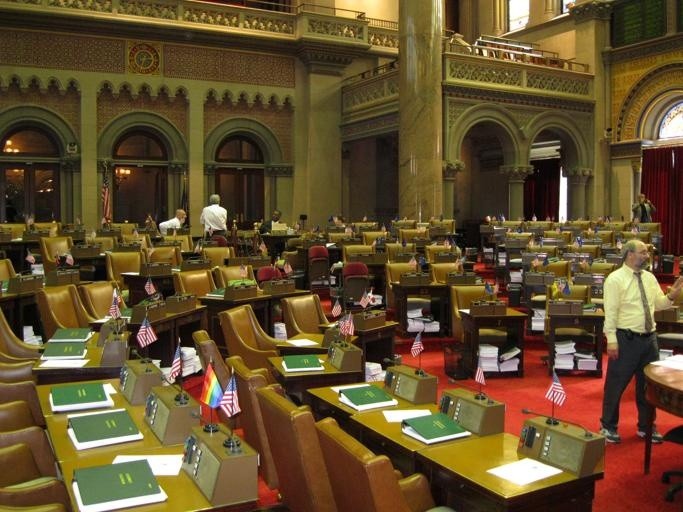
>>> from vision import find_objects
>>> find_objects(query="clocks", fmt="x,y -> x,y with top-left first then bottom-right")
126,40 -> 163,77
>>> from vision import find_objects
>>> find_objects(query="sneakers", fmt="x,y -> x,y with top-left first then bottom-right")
637,431 -> 662,442
599,429 -> 620,443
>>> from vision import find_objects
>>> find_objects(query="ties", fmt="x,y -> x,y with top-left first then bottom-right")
634,272 -> 652,333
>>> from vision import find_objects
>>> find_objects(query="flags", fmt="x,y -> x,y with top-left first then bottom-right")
475,358 -> 486,386
235,212 -> 639,377
144,278 -> 156,296
26,249 -> 34,265
137,317 -> 158,348
101,167 -> 112,225
545,369 -> 566,407
167,344 -> 181,381
199,363 -> 224,408
109,293 -> 121,320
219,374 -> 241,417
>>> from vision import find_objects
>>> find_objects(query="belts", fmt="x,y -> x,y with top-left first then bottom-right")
617,328 -> 653,337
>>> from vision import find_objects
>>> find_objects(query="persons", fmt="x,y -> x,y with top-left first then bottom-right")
632,194 -> 656,224
598,239 -> 683,444
158,209 -> 187,237
200,194 -> 228,241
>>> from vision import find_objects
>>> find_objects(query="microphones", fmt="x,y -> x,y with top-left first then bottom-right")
447,377 -> 496,406
384,357 -> 423,376
160,375 -> 189,404
522,408 -> 592,439
131,349 -> 151,372
192,411 -> 242,455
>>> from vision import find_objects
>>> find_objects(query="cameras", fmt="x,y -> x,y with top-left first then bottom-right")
644,200 -> 650,203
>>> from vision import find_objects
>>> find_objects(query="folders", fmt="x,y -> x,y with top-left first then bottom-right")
40,342 -> 88,359
582,303 -> 598,312
1,280 -> 9,290
282,355 -> 325,372
339,384 -> 398,411
121,308 -> 132,319
71,459 -> 168,512
206,287 -> 224,297
49,382 -> 114,411
47,328 -> 96,342
66,408 -> 144,451
401,413 -> 472,445
